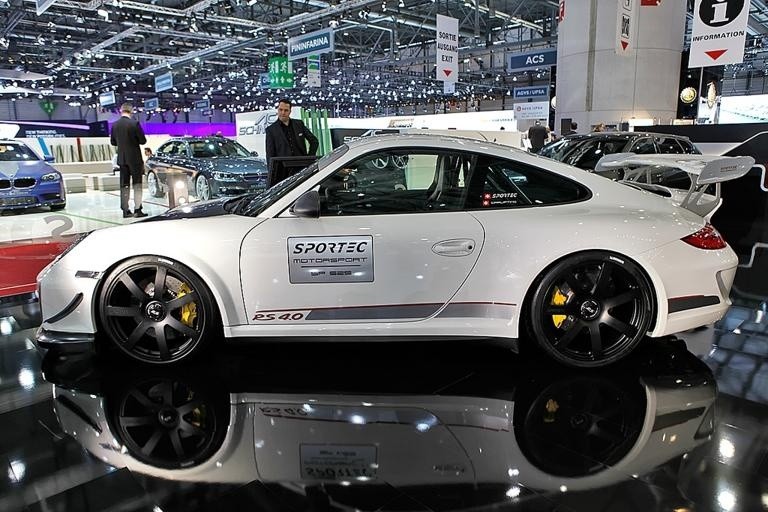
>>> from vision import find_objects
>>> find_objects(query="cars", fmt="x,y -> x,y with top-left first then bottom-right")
34,130 -> 740,378
0,140 -> 66,216
40,350 -> 721,510
144,135 -> 269,202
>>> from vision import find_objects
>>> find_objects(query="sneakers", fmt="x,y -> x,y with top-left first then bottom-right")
123,210 -> 148,218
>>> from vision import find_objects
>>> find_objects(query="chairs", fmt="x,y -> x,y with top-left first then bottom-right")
430,152 -> 452,204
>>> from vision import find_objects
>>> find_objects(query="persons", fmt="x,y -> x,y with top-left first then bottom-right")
528,120 -> 548,148
145,148 -> 152,156
265,99 -> 319,173
563,122 -> 578,136
111,103 -> 148,217
593,122 -> 604,132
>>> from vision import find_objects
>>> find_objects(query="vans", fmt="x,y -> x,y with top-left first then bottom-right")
534,132 -> 704,186
